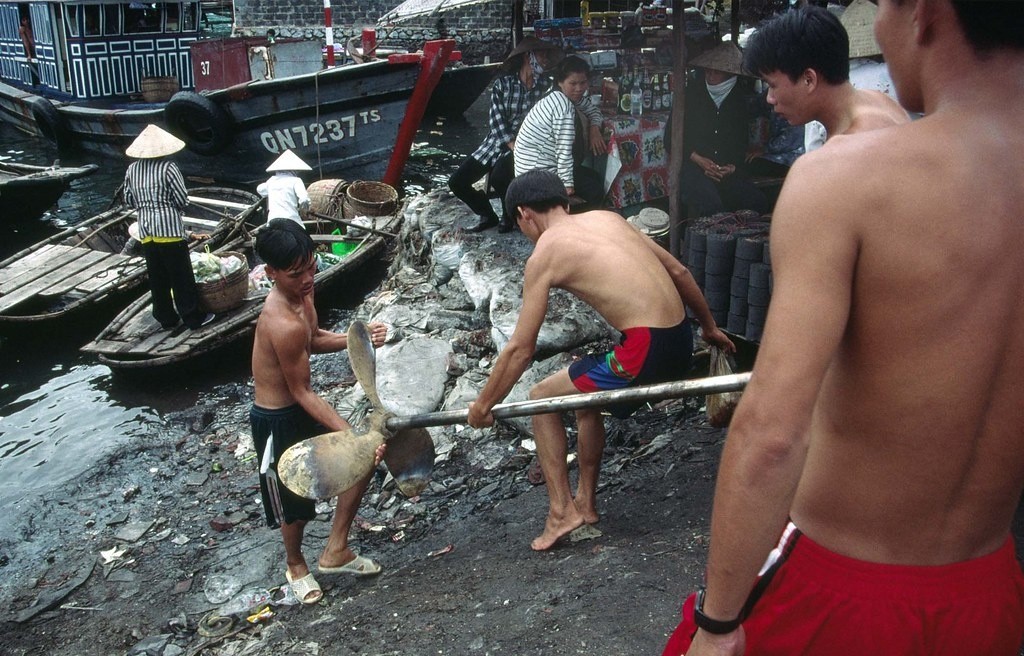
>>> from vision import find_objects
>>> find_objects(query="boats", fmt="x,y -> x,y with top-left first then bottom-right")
0,187 -> 263,324
0,0 -> 505,193
79,178 -> 404,386
1,159 -> 99,222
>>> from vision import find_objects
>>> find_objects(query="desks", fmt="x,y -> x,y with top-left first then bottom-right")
572,99 -> 671,214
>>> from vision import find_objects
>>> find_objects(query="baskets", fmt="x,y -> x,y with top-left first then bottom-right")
195,252 -> 249,314
304,179 -> 399,229
141,77 -> 179,104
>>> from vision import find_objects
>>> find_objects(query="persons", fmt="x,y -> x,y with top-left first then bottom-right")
256,149 -> 313,231
449,35 -> 606,235
740,0 -> 922,215
120,221 -> 211,255
250,217 -> 387,605
123,123 -> 216,332
467,169 -> 736,550
663,0 -> 1024,656
664,40 -> 777,222
19,11 -> 33,60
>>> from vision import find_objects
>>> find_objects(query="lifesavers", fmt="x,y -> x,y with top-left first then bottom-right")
164,91 -> 229,155
31,97 -> 69,151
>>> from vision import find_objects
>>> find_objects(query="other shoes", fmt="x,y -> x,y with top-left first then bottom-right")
200,311 -> 216,326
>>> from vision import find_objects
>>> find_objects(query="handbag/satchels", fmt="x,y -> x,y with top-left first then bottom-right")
197,244 -> 220,276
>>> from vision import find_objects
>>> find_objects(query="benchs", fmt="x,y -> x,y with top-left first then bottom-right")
301,217 -> 350,225
120,207 -> 220,229
185,196 -> 249,210
309,230 -> 347,240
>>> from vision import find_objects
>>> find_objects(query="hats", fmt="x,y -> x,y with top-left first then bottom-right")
126,123 -> 185,159
128,222 -> 140,242
686,40 -> 759,76
266,149 -> 311,172
838,0 -> 885,57
502,35 -> 565,72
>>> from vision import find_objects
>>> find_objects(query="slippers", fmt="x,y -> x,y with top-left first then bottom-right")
284,568 -> 323,604
318,553 -> 382,574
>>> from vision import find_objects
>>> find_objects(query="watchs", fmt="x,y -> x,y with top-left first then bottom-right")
694,588 -> 744,633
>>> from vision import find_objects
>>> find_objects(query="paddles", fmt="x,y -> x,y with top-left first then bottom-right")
96,181 -> 125,225
298,208 -> 397,238
189,202 -> 258,228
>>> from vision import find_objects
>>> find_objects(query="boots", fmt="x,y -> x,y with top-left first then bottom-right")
465,190 -> 499,232
498,194 -> 515,233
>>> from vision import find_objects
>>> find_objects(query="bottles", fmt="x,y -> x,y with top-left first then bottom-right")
580,0 -> 589,26
616,64 -> 672,116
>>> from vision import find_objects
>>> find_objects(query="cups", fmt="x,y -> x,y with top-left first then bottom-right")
619,11 -> 635,27
591,95 -> 601,105
589,12 -> 603,28
604,11 -> 619,28
